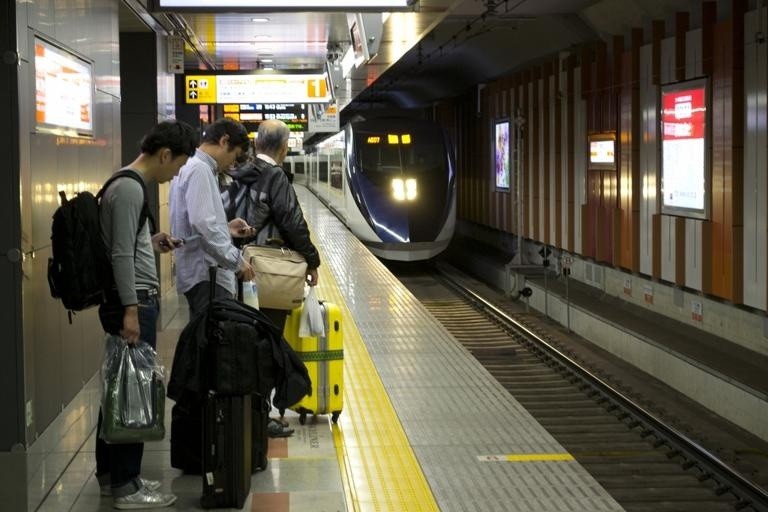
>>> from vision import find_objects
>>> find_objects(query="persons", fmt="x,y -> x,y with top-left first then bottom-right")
93,120 -> 200,509
237,119 -> 319,437
165,115 -> 256,325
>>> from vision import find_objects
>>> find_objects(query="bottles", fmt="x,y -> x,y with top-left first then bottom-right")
242,279 -> 260,311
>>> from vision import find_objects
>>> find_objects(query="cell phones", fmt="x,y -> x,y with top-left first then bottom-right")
237,223 -> 262,233
159,237 -> 185,248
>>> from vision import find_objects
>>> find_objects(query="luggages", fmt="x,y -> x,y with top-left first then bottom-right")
280,279 -> 344,425
171,266 -> 272,510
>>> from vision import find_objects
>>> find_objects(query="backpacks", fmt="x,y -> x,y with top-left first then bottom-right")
46,169 -> 149,311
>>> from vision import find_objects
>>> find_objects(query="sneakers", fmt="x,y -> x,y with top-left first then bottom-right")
113,486 -> 177,509
266,416 -> 294,436
100,478 -> 161,496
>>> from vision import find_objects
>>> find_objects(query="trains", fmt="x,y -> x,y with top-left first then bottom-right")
278,113 -> 458,262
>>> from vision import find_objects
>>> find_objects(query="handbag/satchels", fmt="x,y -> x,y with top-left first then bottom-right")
238,237 -> 309,310
